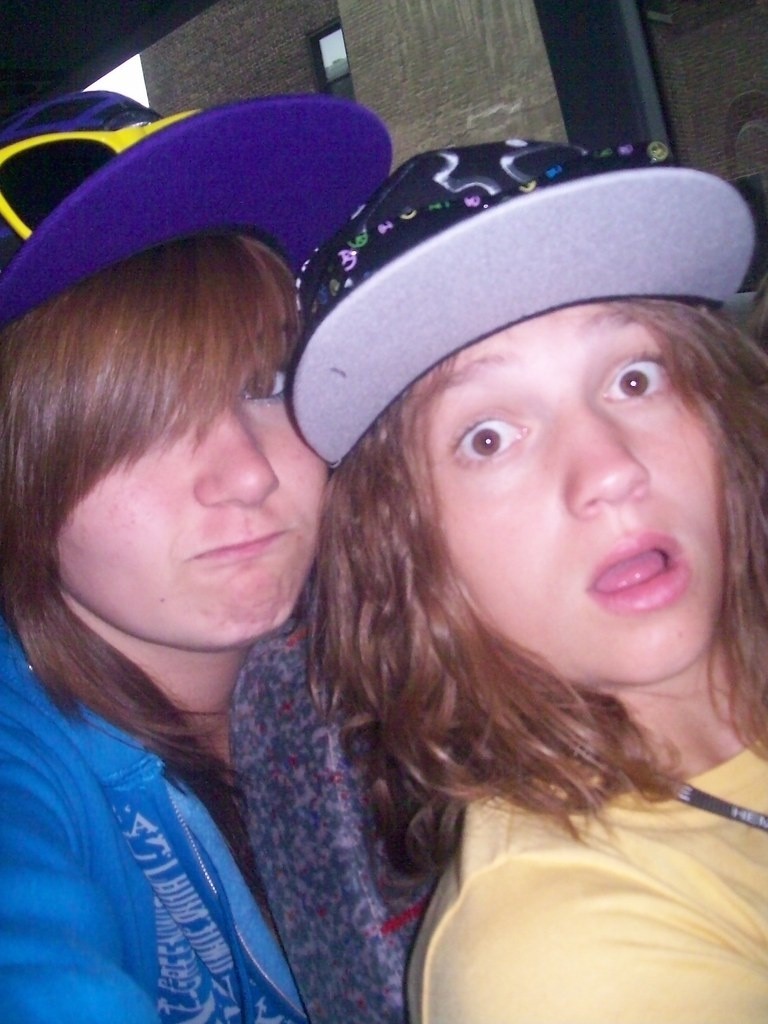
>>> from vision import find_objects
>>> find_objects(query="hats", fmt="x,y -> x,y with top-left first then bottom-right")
0,87 -> 392,332
284,137 -> 758,468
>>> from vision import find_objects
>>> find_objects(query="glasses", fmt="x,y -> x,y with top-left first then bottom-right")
0,108 -> 204,241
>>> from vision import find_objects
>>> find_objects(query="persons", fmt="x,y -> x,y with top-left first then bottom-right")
285,136 -> 768,1024
1,88 -> 463,1024
